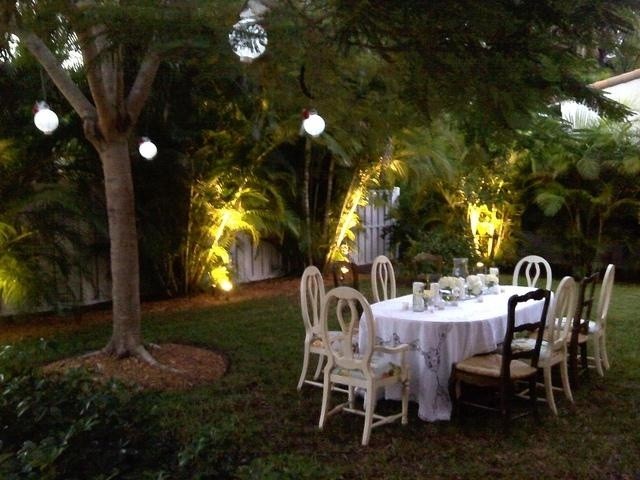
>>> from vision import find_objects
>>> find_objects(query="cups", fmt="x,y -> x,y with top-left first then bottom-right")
491,268 -> 499,278
453,258 -> 469,278
395,300 -> 409,312
412,274 -> 498,313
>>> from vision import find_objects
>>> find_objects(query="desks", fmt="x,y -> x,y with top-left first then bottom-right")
359,283 -> 555,427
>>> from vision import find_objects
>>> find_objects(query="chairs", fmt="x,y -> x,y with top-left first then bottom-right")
369,255 -> 396,302
448,287 -> 553,441
553,263 -> 615,378
529,273 -> 598,393
295,265 -> 351,395
511,255 -> 552,291
312,286 -> 411,447
507,274 -> 577,416
